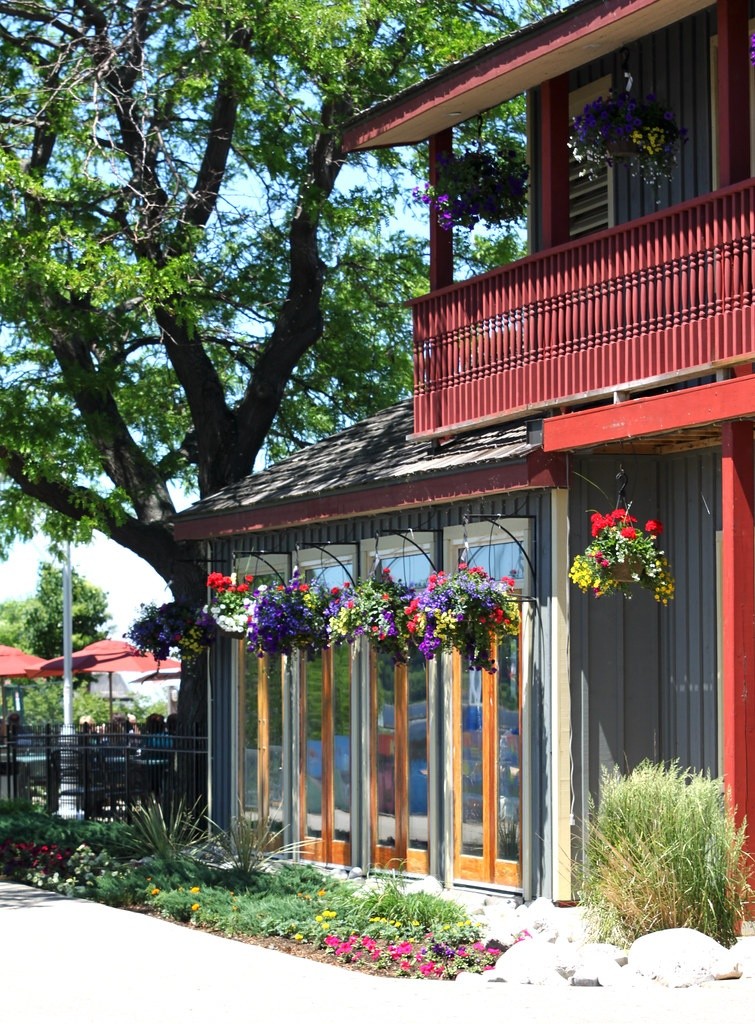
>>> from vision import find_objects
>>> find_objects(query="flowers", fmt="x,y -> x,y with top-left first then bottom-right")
750,33 -> 755,67
412,140 -> 532,233
194,562 -> 521,674
567,86 -> 691,206
567,509 -> 675,607
122,596 -> 215,678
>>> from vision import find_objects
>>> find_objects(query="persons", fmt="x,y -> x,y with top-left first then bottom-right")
6,713 -> 25,746
80,713 -> 178,785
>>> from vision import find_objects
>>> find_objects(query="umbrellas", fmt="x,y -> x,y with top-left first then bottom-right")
0,644 -> 62,718
25,639 -> 183,719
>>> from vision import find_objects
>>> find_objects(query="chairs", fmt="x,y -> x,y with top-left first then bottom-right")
50,747 -> 158,822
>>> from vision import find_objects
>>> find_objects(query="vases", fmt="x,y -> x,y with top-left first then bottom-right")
607,555 -> 644,583
215,625 -> 246,640
606,140 -> 638,158
